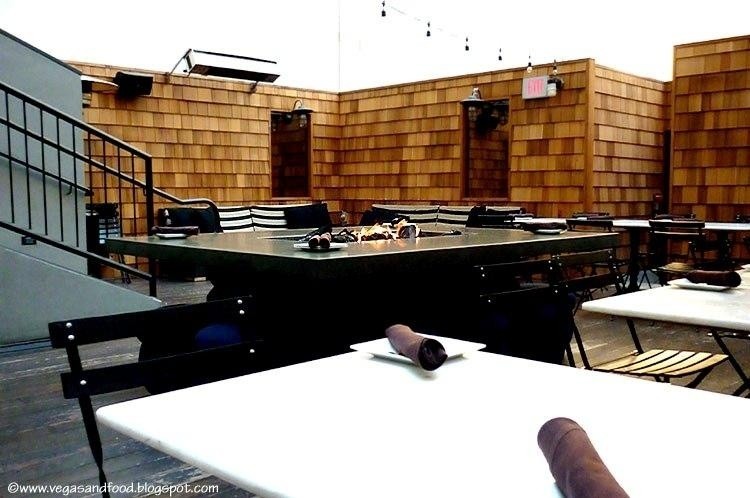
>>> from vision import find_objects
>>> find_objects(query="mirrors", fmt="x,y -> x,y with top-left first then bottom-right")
270,109 -> 313,201
459,97 -> 512,203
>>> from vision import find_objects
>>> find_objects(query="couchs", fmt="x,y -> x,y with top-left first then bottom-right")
358,204 -> 526,228
158,201 -> 329,283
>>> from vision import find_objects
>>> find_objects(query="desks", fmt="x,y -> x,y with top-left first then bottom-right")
93,345 -> 750,498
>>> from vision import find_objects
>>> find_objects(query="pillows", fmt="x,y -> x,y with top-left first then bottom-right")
198,206 -> 223,232
365,208 -> 395,226
466,208 -> 486,227
284,202 -> 332,229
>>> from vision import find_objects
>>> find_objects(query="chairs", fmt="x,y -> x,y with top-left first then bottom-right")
471,213 -> 750,383
86,203 -> 132,284
47,297 -> 270,498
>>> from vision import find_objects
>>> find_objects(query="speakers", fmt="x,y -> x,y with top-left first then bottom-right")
116,71 -> 153,96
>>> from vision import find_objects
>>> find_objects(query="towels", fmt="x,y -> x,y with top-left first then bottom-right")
686,269 -> 741,288
387,323 -> 447,371
308,232 -> 331,248
537,418 -> 629,498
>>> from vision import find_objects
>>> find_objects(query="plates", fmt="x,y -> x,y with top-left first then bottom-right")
534,224 -> 567,235
155,231 -> 192,240
666,278 -> 740,294
351,333 -> 488,367
294,242 -> 350,254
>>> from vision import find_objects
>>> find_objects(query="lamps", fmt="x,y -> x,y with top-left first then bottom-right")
460,87 -> 484,121
490,101 -> 509,125
283,100 -> 312,128
527,55 -> 558,75
